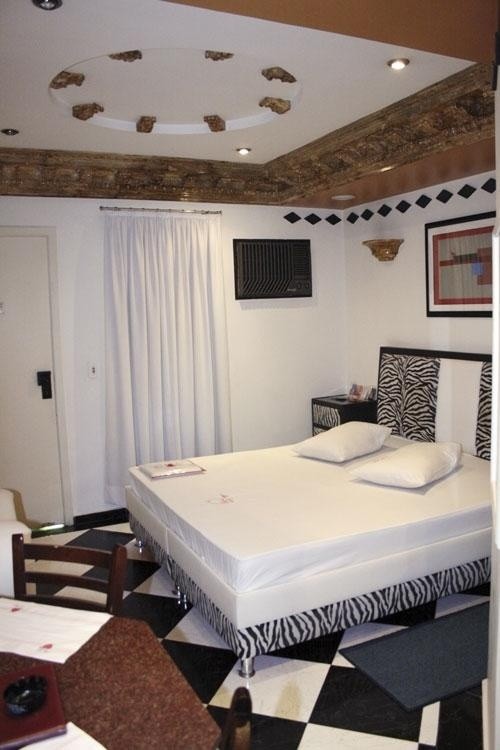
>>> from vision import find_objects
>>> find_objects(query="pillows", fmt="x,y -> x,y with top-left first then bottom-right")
290,418 -> 464,490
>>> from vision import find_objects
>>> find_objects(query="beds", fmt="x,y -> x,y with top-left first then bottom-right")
123,346 -> 492,680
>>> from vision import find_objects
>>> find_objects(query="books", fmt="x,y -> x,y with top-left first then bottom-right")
1,663 -> 67,750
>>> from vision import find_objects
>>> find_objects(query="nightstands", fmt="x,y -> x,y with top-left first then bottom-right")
310,393 -> 379,439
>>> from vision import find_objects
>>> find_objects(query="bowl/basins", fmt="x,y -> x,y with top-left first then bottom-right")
2,674 -> 48,717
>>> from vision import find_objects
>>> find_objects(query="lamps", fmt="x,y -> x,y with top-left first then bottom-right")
361,236 -> 404,263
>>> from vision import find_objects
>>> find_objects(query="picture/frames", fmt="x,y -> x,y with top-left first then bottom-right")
424,210 -> 496,318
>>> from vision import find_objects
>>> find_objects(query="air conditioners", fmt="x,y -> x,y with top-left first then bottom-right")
230,238 -> 313,301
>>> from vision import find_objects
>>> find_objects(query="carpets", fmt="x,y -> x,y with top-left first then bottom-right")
338,599 -> 489,714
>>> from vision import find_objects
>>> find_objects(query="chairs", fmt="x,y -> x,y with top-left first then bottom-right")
7,531 -> 128,616
